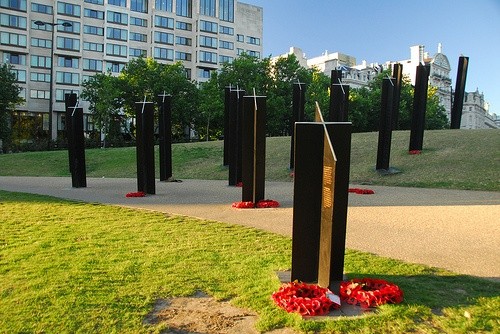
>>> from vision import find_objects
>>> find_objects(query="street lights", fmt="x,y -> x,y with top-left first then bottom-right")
34,19 -> 73,150
